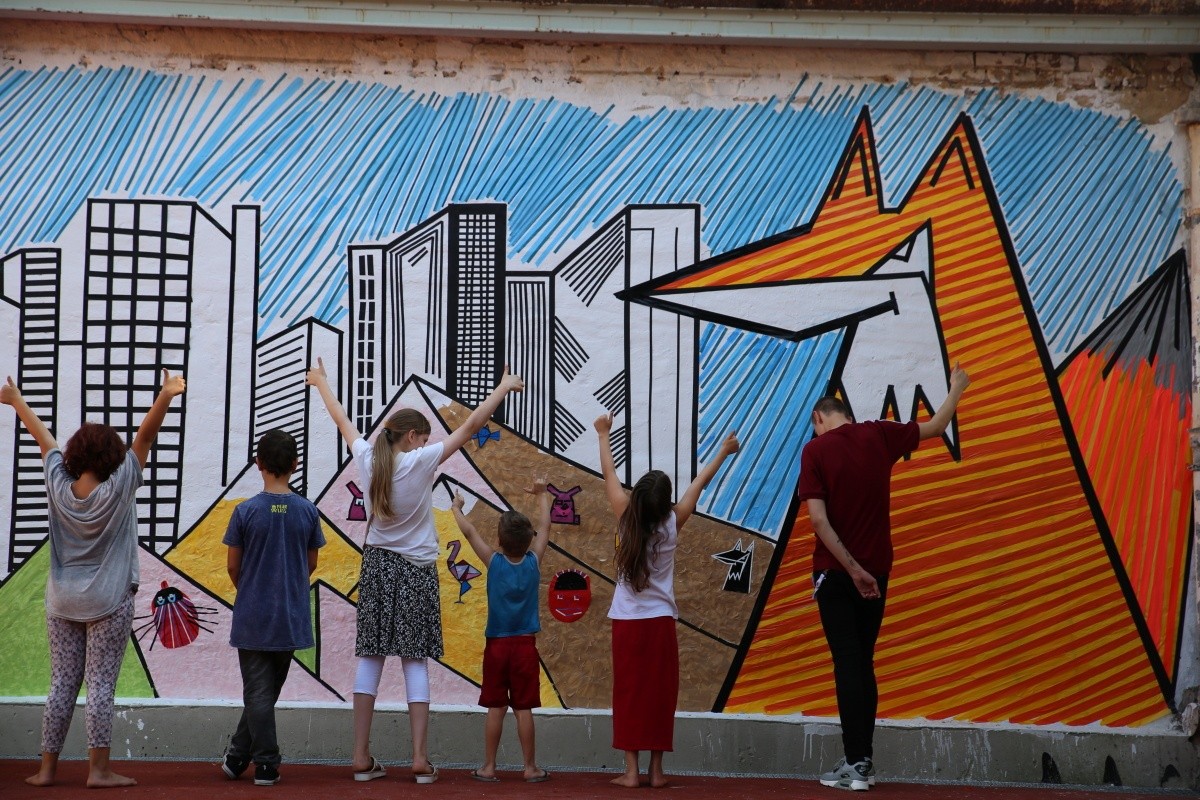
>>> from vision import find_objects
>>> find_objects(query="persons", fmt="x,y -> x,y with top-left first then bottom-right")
306,356 -> 524,784
797,360 -> 970,790
594,413 -> 738,786
0,368 -> 187,787
452,471 -> 552,782
221,429 -> 327,786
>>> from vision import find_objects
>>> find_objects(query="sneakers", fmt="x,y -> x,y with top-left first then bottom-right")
254,764 -> 280,785
819,756 -> 869,790
864,757 -> 875,785
221,753 -> 246,778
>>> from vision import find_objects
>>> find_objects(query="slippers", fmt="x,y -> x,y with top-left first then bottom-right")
472,770 -> 496,782
354,757 -> 386,781
416,761 -> 439,783
526,769 -> 551,782
